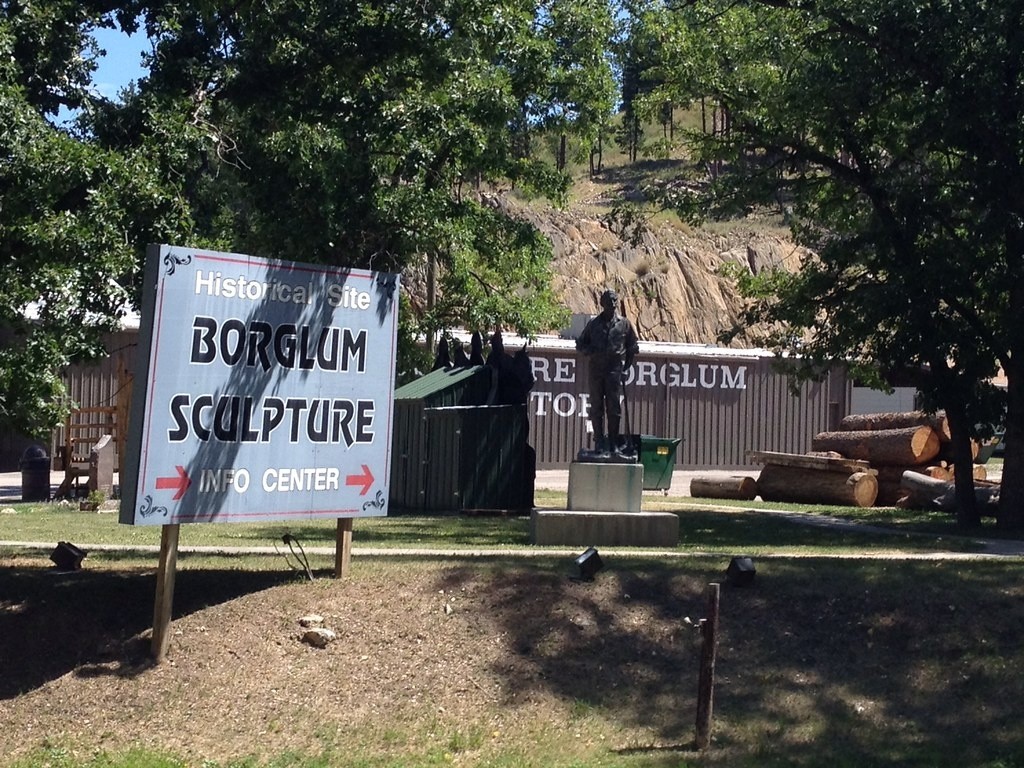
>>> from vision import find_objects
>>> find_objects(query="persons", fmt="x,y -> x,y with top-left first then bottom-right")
575,288 -> 639,456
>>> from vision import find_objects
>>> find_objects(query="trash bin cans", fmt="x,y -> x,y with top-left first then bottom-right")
19,444 -> 51,502
593,434 -> 681,490
